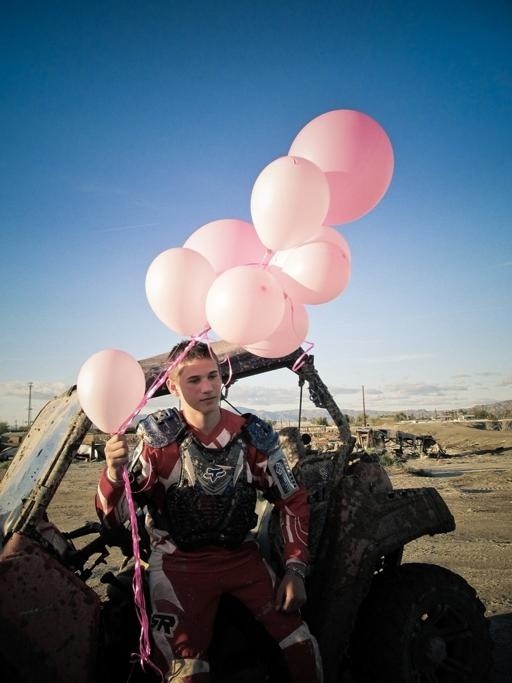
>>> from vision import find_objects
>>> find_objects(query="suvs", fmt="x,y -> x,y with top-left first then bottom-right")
0,330 -> 494,683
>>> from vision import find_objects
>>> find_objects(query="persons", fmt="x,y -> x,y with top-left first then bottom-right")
93,339 -> 325,683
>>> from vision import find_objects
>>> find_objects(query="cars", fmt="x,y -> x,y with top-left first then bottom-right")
0,441 -> 20,463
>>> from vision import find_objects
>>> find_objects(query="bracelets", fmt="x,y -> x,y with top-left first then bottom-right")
287,564 -> 307,581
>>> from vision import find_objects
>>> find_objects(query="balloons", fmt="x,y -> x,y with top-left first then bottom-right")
75,348 -> 145,436
143,108 -> 396,359
